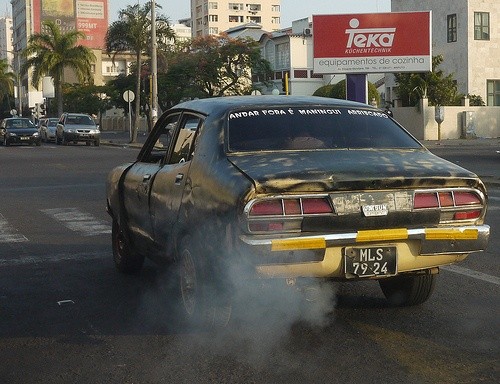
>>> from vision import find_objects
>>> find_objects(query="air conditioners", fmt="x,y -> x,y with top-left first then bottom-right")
303,28 -> 312,34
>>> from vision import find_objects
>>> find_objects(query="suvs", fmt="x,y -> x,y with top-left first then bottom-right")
37,113 -> 102,146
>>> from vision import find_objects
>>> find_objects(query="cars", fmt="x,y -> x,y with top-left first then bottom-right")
0,118 -> 41,146
105,95 -> 491,324
